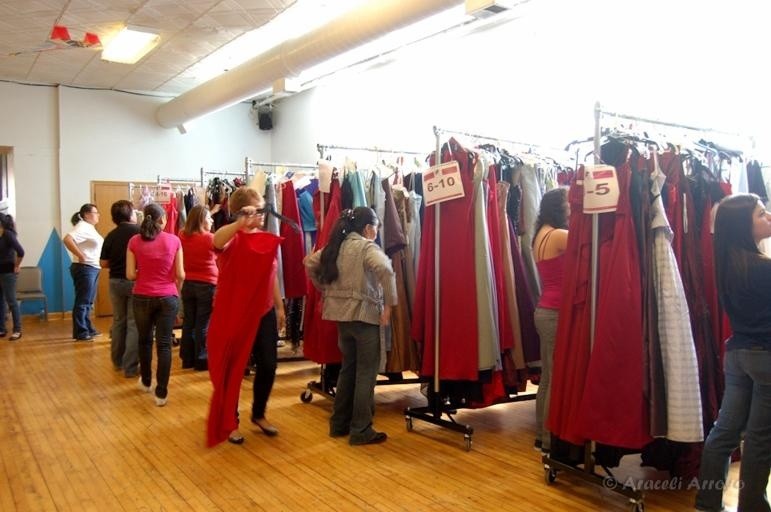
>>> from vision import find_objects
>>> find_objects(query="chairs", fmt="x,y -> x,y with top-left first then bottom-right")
15,267 -> 47,321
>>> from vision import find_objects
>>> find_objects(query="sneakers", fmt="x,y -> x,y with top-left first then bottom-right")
76,335 -> 97,342
89,330 -> 101,336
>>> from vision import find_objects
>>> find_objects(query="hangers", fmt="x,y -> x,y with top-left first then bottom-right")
137,184 -> 146,202
175,184 -> 185,197
266,166 -> 286,191
184,184 -> 193,195
378,161 -> 397,184
205,175 -> 232,194
425,136 -> 568,178
414,157 -> 420,168
565,113 -> 742,161
239,204 -> 300,234
220,174 -> 238,191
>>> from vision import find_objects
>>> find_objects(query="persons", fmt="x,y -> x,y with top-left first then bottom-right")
125,201 -> 187,407
61,202 -> 106,342
688,189 -> 771,512
99,198 -> 142,376
0,212 -> 25,342
529,183 -> 572,455
177,202 -> 223,373
301,203 -> 398,447
211,185 -> 288,445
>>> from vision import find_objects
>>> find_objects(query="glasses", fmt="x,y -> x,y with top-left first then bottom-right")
87,211 -> 100,216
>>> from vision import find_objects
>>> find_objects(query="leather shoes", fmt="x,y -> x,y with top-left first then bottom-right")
0,331 -> 8,337
251,415 -> 279,435
365,432 -> 387,444
9,333 -> 22,341
229,429 -> 243,444
153,387 -> 168,406
139,376 -> 153,392
